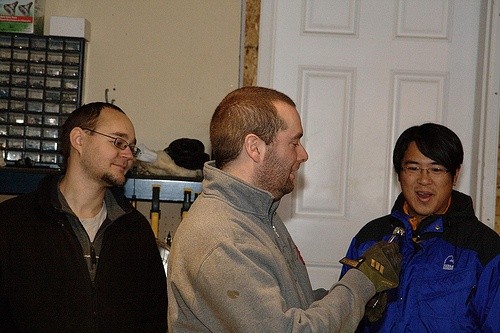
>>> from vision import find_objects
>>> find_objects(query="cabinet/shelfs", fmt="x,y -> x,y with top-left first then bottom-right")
0,32 -> 85,173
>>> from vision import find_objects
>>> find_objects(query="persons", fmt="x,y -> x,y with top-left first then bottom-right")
338,122 -> 500,333
166,84 -> 407,333
0,101 -> 168,333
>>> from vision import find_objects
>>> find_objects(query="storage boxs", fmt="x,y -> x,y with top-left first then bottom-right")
0,0 -> 45,36
50,16 -> 90,42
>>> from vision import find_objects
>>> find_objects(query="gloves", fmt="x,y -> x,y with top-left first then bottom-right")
338,240 -> 403,292
365,290 -> 388,322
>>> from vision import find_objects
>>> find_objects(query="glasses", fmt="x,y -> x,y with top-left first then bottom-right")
404,165 -> 449,176
82,127 -> 141,156
